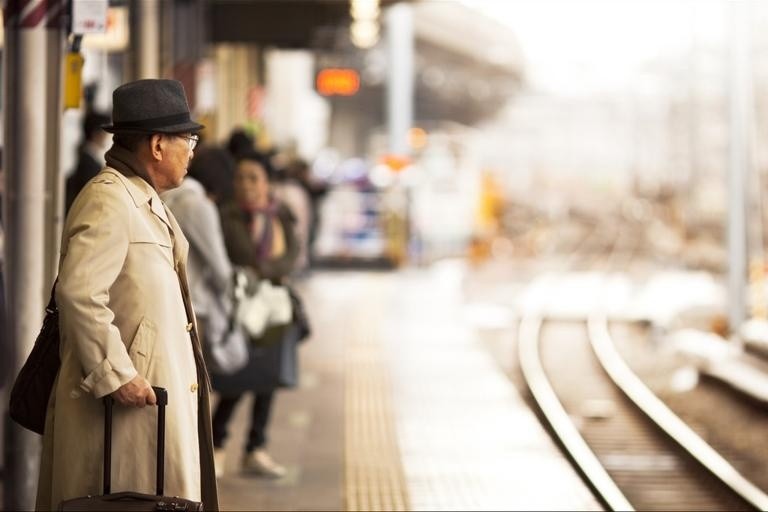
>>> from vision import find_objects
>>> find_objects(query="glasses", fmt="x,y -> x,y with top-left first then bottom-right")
168,132 -> 199,150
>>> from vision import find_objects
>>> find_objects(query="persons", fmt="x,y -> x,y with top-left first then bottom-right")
68,113 -> 112,209
161,130 -> 333,477
36,77 -> 220,511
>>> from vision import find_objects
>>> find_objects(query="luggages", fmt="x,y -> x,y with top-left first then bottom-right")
56,385 -> 208,512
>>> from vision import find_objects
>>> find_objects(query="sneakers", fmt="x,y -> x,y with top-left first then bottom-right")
236,450 -> 286,480
214,449 -> 228,483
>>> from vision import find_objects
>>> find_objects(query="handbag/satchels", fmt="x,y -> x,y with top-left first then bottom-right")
6,275 -> 61,438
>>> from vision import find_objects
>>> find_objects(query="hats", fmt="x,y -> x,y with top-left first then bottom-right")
99,76 -> 206,135
